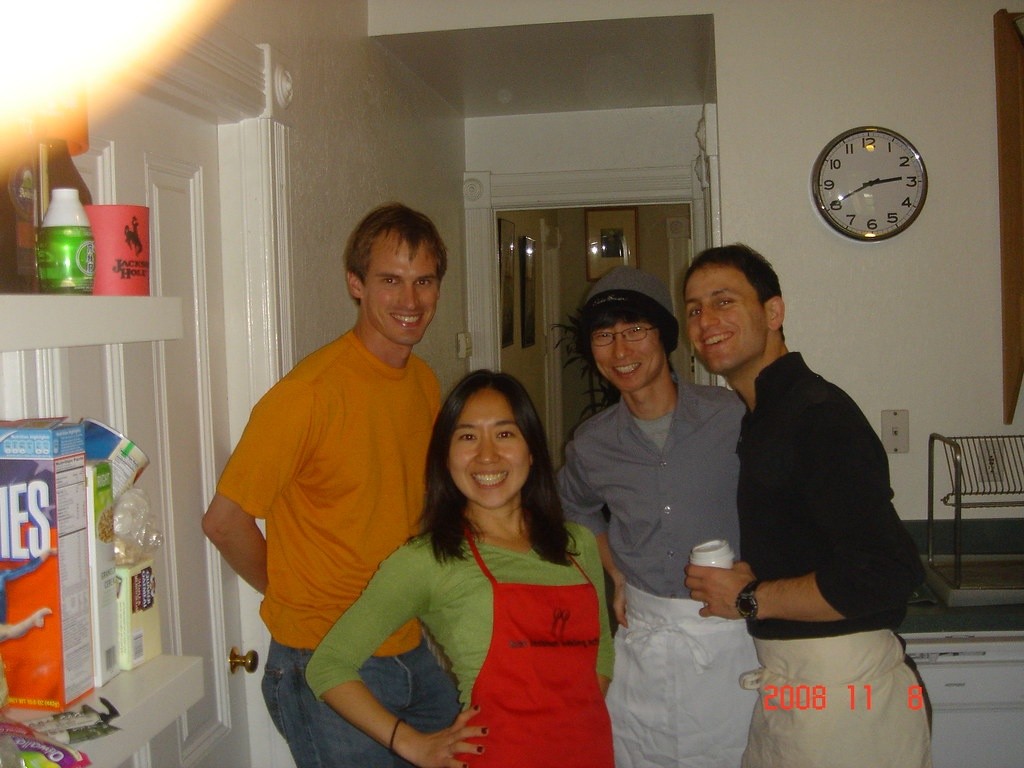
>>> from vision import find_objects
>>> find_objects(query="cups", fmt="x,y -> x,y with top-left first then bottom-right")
690,539 -> 735,570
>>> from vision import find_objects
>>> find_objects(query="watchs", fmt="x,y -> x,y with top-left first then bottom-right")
737,577 -> 758,620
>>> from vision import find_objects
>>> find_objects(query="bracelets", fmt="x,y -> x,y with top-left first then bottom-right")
389,718 -> 408,751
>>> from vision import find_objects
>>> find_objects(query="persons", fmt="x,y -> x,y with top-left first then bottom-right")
553,263 -> 762,768
202,203 -> 464,768
304,368 -> 615,768
684,244 -> 934,768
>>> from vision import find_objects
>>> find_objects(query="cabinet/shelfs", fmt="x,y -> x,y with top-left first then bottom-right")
0,294 -> 205,768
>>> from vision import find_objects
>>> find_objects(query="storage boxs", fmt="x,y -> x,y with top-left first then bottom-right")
116,554 -> 162,672
0,414 -> 94,712
85,457 -> 120,687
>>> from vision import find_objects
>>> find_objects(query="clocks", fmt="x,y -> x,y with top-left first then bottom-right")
811,126 -> 929,242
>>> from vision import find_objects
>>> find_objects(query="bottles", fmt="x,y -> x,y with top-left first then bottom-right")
38,188 -> 95,294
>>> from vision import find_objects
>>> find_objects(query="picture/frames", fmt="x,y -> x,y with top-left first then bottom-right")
499,218 -> 516,349
584,206 -> 640,282
521,235 -> 536,348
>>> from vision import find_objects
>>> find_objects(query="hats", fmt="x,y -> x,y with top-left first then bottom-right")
582,265 -> 679,352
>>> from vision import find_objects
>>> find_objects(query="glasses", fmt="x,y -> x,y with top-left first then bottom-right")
590,325 -> 657,346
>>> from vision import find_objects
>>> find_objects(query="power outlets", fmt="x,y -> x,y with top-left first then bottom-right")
883,410 -> 910,454
